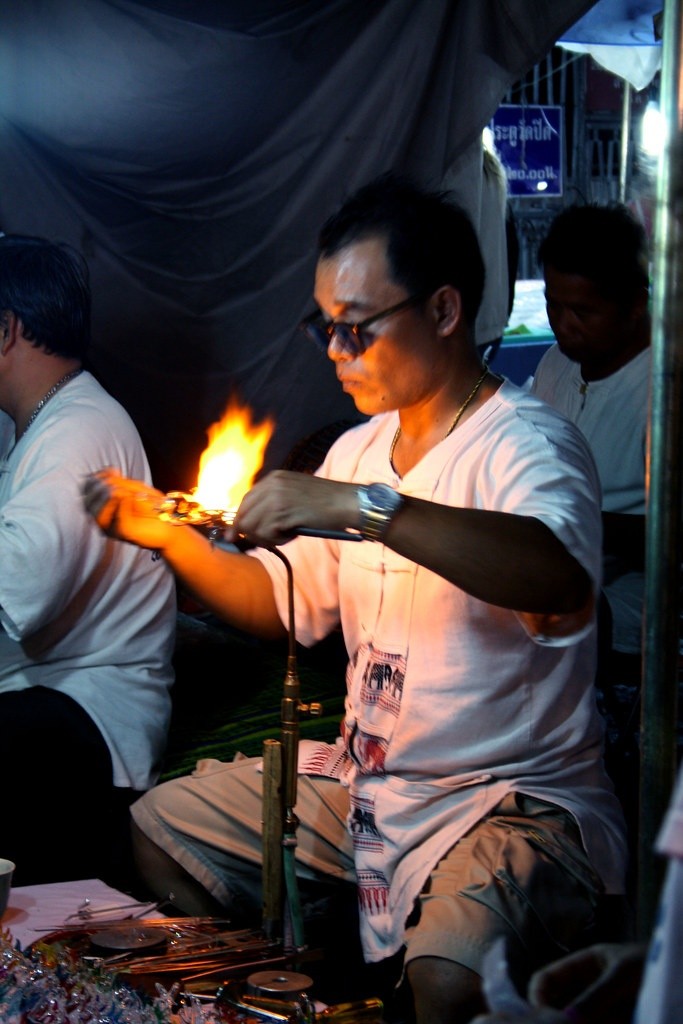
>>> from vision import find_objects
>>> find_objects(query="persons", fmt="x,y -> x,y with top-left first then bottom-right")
532,201 -> 652,684
81,179 -> 629,1024
0,233 -> 177,886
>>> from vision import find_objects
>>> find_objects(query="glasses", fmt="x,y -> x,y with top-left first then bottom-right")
295,290 -> 436,357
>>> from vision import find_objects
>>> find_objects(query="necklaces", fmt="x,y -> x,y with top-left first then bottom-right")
389,368 -> 489,463
22,371 -> 79,434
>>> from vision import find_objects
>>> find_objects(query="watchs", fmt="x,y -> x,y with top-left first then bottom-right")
359,482 -> 402,541
